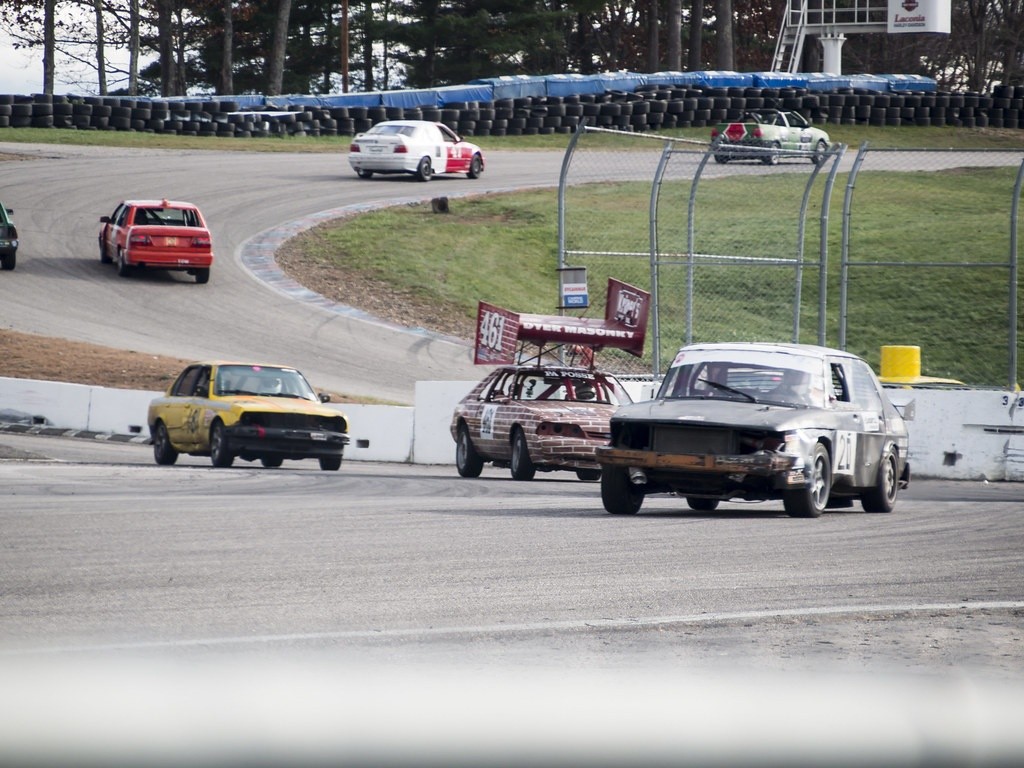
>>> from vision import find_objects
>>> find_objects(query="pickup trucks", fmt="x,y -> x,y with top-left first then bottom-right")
708,107 -> 833,167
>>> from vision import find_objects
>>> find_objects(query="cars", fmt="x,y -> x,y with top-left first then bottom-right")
148,361 -> 352,472
97,198 -> 215,282
347,118 -> 487,182
449,363 -> 637,480
0,200 -> 19,271
593,340 -> 912,519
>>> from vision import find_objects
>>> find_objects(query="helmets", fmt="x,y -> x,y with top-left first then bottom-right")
263,377 -> 282,393
575,384 -> 596,401
783,370 -> 812,395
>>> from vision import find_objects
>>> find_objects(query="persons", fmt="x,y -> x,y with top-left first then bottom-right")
764,369 -> 820,407
767,113 -> 781,125
566,383 -> 598,401
258,378 -> 282,394
